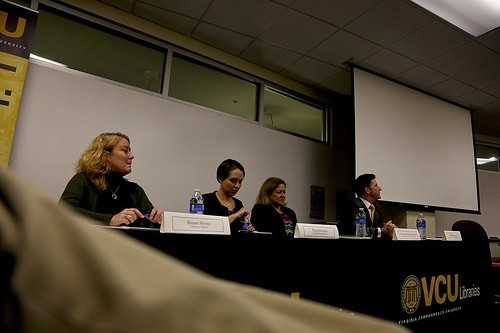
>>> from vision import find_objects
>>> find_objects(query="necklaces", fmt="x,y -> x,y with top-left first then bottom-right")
218,193 -> 231,207
106,183 -> 121,200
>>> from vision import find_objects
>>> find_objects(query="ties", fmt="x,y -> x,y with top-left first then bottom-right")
369,204 -> 375,225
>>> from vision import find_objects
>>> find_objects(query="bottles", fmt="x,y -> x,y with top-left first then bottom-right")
416,214 -> 427,239
189,189 -> 205,215
355,208 -> 366,237
239,215 -> 251,230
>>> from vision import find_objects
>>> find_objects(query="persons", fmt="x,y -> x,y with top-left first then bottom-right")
57,133 -> 163,229
202,159 -> 255,235
340,173 -> 398,237
250,177 -> 298,236
